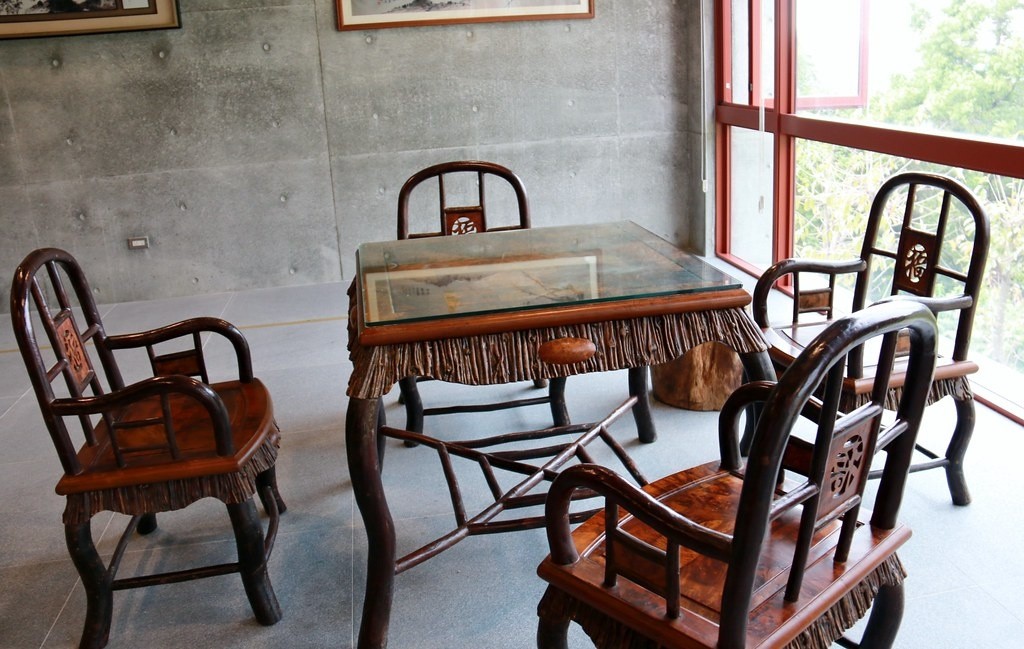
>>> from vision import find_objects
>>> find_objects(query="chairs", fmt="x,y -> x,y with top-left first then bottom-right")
397,160 -> 572,452
536,304 -> 937,649
740,171 -> 993,506
12,246 -> 283,649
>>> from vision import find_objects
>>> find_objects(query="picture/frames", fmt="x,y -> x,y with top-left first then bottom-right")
362,246 -> 605,322
0,0 -> 182,39
334,0 -> 596,32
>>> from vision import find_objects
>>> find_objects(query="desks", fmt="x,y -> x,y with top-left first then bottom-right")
346,220 -> 776,649
